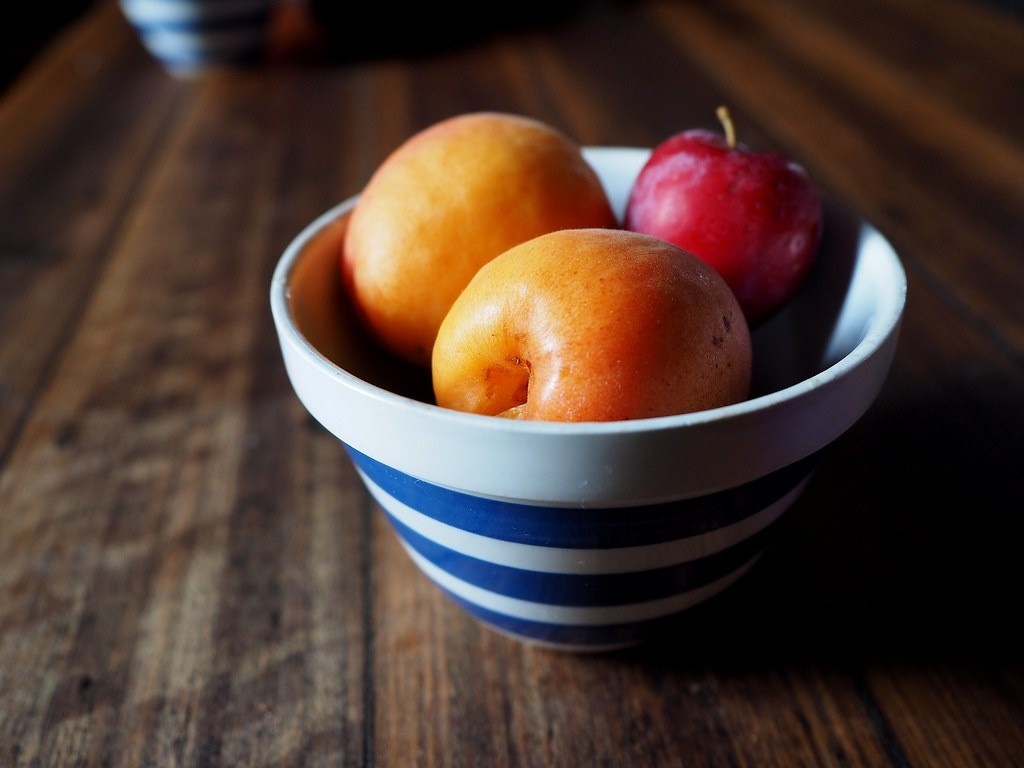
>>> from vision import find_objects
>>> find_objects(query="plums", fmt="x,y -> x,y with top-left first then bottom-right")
622,104 -> 823,308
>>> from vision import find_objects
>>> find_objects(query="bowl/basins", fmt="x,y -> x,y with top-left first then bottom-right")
116,0 -> 279,80
269,144 -> 909,653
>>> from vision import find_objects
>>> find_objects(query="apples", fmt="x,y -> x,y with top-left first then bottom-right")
344,112 -> 620,356
431,232 -> 750,425
